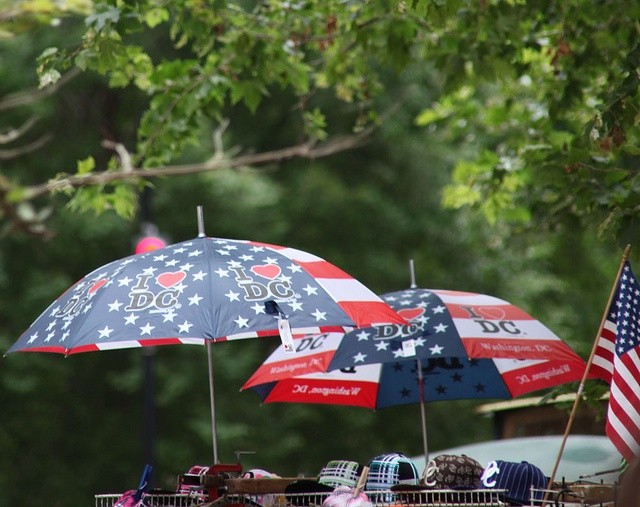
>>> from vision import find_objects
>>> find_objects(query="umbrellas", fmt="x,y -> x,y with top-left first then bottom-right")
3,205 -> 410,354
238,259 -> 589,410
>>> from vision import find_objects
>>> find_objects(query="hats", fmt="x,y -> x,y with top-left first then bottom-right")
283,459 -> 366,504
452,461 -> 549,505
391,455 -> 486,500
364,452 -> 419,500
223,471 -> 284,506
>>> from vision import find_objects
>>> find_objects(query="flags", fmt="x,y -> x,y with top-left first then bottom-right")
583,256 -> 639,469
